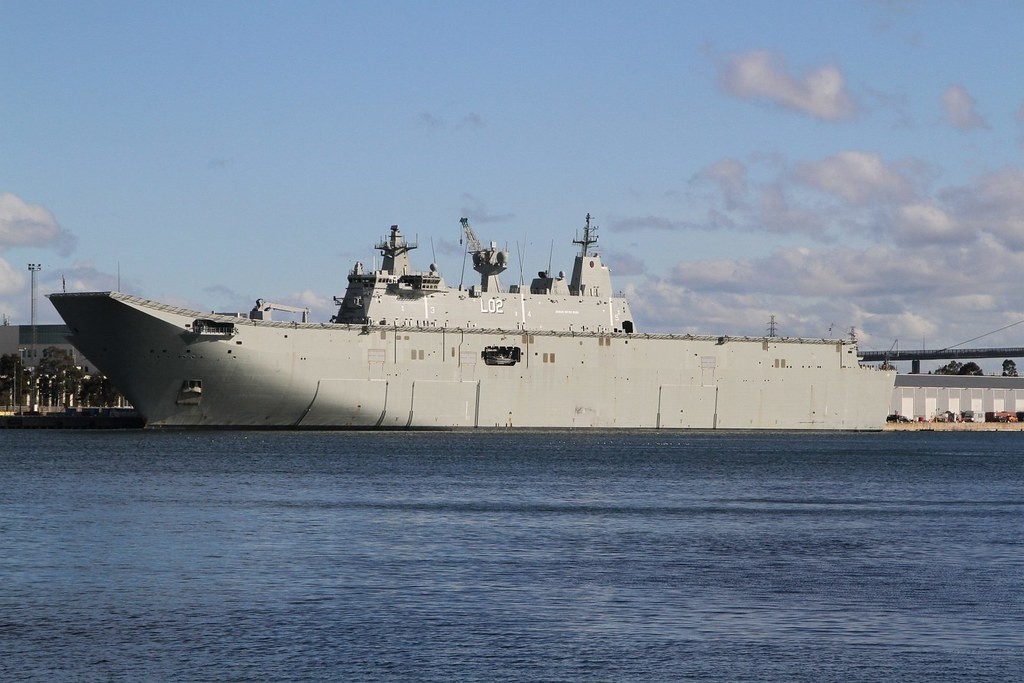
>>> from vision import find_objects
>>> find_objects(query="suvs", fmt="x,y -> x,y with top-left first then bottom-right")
1006,415 -> 1018,422
887,414 -> 908,424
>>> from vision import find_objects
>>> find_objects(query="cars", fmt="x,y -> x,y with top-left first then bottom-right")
962,415 -> 974,422
992,416 -> 1007,422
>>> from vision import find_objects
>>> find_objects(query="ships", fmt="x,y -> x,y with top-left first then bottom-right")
45,212 -> 899,432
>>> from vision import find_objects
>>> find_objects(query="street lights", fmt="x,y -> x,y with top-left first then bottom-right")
28,263 -> 41,414
